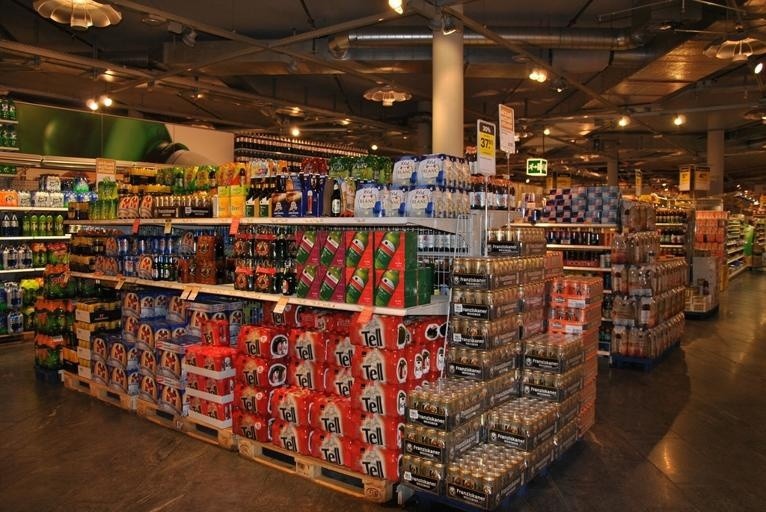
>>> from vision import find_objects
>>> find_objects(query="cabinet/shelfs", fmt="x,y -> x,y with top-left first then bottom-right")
0,182 -> 766,510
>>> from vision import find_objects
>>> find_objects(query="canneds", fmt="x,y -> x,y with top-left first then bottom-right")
153,196 -> 212,207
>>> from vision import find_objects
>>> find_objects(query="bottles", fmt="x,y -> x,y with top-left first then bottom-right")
234,131 -> 368,162
295,230 -> 403,309
295,153 -> 517,217
609,207 -> 690,357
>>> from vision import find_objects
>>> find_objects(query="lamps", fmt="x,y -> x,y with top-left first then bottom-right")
746,54 -> 764,76
